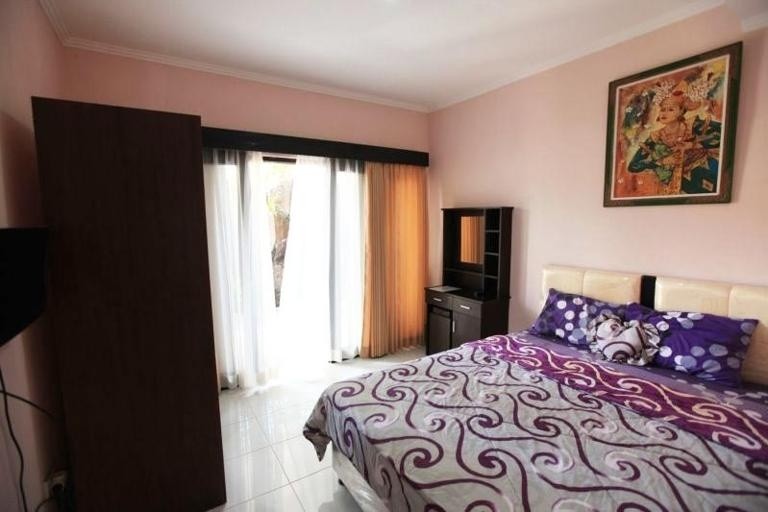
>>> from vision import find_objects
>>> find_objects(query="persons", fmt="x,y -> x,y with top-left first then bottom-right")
627,74 -> 722,195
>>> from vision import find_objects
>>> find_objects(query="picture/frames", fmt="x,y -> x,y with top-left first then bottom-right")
600,40 -> 744,209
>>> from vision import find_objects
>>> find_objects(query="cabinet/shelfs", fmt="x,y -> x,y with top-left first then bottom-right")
423,207 -> 513,354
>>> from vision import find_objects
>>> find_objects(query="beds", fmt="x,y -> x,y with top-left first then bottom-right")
311,267 -> 767,512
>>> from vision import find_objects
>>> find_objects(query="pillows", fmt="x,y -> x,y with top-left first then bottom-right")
531,289 -> 761,387
